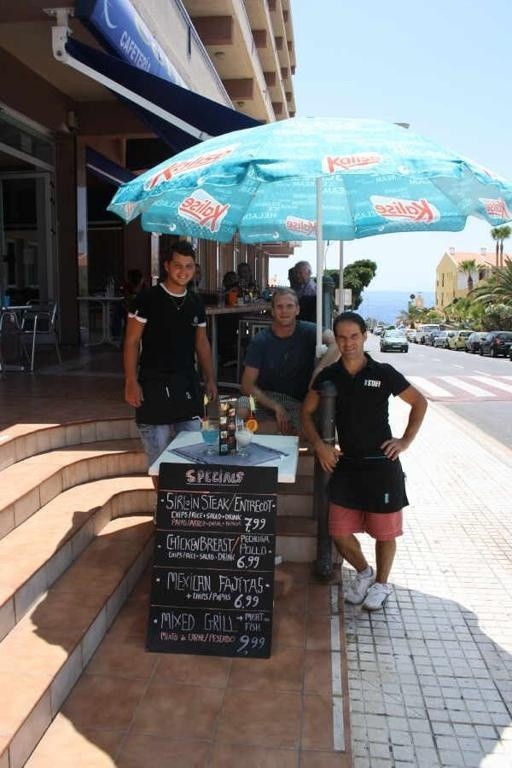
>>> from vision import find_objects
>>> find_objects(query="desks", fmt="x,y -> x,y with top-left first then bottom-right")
0,296 -> 300,567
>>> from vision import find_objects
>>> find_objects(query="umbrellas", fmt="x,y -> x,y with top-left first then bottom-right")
102,107 -> 512,584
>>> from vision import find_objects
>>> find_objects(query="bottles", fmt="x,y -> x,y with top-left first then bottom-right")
219,280 -> 271,307
119,285 -> 125,296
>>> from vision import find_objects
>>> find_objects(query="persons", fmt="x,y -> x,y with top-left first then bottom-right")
185,259 -> 317,307
298,309 -> 429,611
119,241 -> 213,526
236,288 -> 341,442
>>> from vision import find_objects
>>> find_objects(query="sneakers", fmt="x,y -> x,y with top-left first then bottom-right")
345,565 -> 376,604
222,360 -> 237,367
362,582 -> 394,611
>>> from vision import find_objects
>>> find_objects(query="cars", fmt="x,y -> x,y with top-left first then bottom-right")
368,324 -> 475,353
464,332 -> 488,353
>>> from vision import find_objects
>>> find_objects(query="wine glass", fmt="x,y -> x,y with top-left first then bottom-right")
235,423 -> 253,456
201,421 -> 219,456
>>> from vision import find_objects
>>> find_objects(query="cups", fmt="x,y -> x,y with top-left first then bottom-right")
4,295 -> 10,307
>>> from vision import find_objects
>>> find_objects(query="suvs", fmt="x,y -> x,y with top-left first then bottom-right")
480,331 -> 512,358
